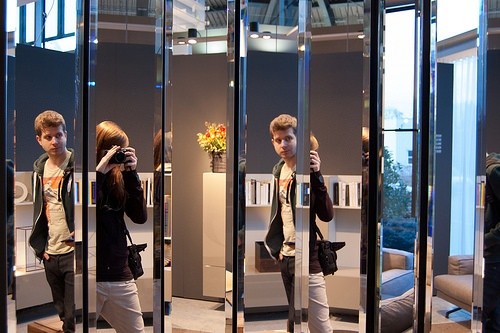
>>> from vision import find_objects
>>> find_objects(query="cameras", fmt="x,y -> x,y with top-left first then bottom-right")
110,149 -> 130,164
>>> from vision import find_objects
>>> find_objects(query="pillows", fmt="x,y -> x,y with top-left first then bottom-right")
380,288 -> 414,333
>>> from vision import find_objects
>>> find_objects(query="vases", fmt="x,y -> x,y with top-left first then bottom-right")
212,148 -> 227,172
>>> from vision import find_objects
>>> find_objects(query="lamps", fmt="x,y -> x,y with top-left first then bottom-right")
249,20 -> 259,37
187,26 -> 198,46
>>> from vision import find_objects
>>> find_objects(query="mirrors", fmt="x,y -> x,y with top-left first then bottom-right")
0,0 -> 500,333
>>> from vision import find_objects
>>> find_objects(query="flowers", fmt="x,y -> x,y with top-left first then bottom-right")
198,120 -> 229,152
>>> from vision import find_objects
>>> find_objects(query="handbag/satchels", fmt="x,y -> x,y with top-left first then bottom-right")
317,239 -> 346,277
126,243 -> 147,280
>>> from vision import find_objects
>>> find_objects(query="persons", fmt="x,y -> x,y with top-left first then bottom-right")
28,111 -> 74,333
264,114 -> 334,333
61,121 -> 148,333
481,153 -> 500,333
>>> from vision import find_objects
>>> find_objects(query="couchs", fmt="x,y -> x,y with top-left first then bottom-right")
380,248 -> 415,300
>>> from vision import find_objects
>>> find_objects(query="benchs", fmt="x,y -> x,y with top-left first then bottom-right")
434,255 -> 474,319
